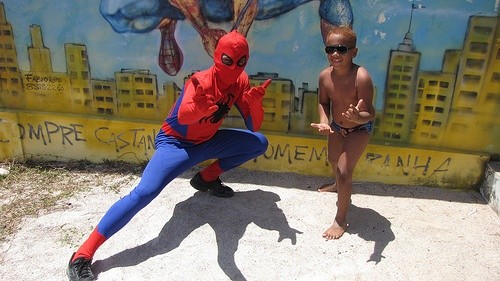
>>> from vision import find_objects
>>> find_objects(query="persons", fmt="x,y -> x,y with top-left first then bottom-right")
65,29 -> 272,281
310,27 -> 375,239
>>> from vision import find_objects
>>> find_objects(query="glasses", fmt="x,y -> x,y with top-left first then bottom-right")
325,45 -> 353,54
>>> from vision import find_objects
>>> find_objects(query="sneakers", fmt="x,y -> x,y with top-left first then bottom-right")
190,172 -> 234,198
66,251 -> 95,281
